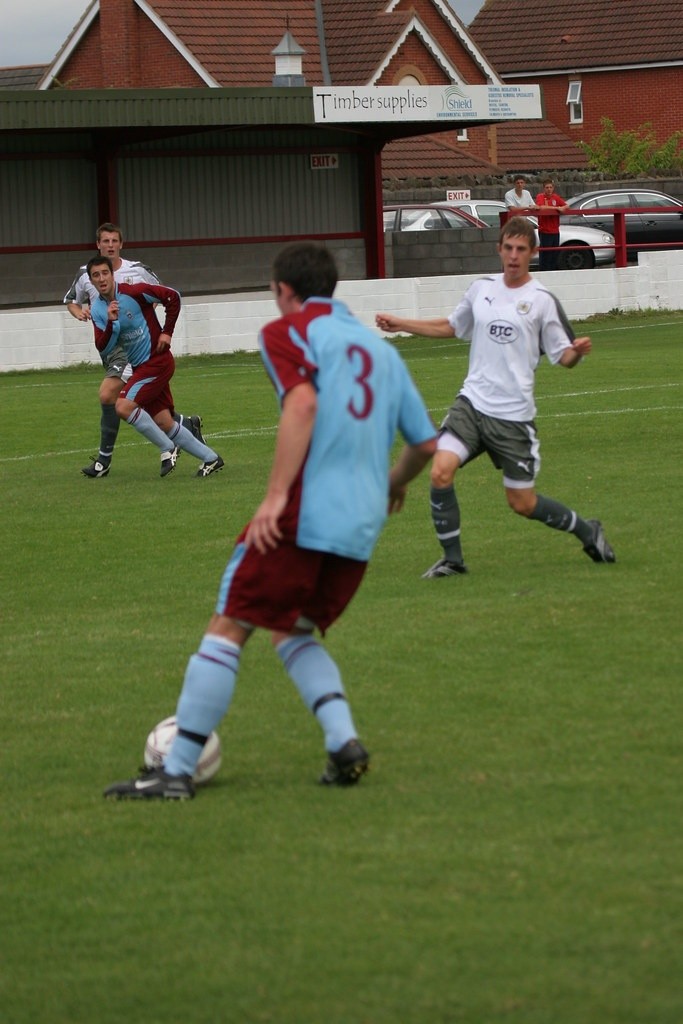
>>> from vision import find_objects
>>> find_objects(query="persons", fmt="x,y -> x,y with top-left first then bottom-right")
376,217 -> 616,575
87,255 -> 226,479
505,175 -> 540,221
536,179 -> 569,271
63,223 -> 207,478
104,242 -> 438,801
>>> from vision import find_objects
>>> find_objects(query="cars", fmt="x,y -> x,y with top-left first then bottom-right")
558,189 -> 683,255
383,207 -> 492,231
399,199 -> 618,272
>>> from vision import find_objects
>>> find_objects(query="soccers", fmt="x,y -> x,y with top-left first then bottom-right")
144,715 -> 223,787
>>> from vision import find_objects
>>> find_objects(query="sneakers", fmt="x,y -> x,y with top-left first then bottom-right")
80,455 -> 110,477
320,739 -> 367,785
197,455 -> 224,478
105,763 -> 196,801
160,445 -> 181,477
421,558 -> 466,578
581,519 -> 616,562
187,414 -> 206,445
